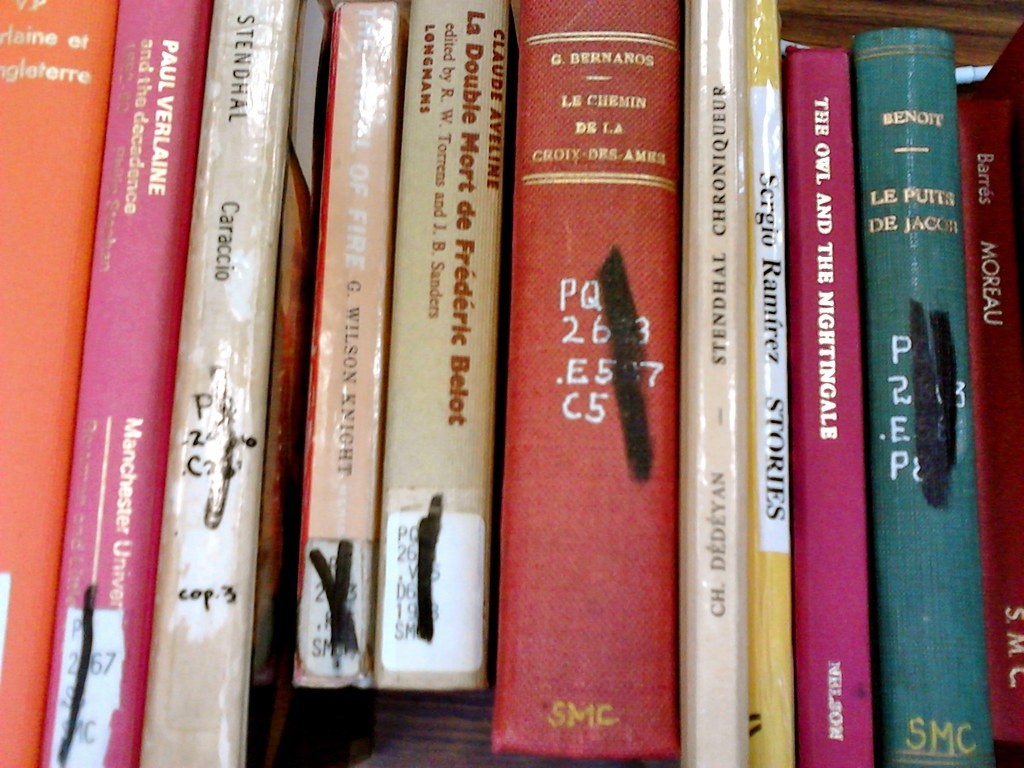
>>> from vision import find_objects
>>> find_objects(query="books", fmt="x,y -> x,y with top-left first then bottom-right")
0,0 -> 1024,768
